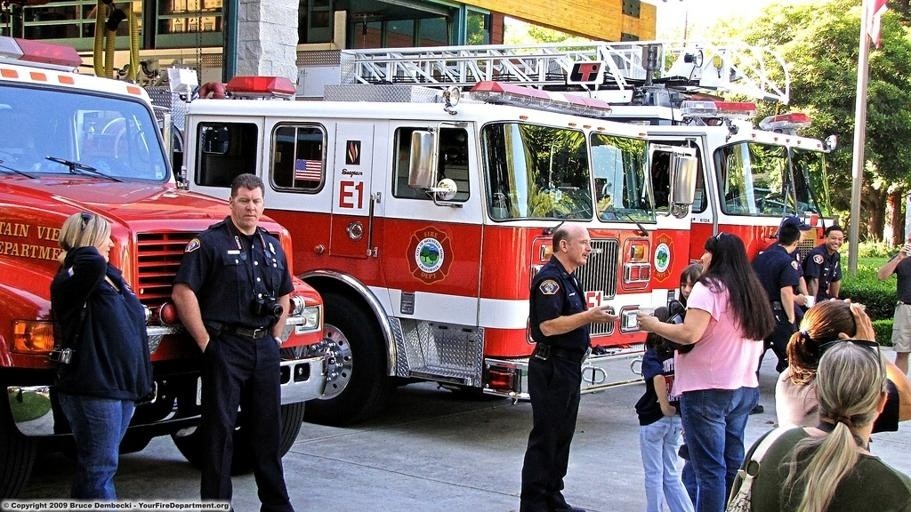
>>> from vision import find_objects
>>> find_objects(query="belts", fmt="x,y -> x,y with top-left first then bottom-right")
534,344 -> 592,365
205,318 -> 274,340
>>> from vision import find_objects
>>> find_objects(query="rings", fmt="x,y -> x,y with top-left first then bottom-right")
637,321 -> 641,326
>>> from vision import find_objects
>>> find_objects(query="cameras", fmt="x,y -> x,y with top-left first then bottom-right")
49,349 -> 73,365
254,296 -> 283,318
141,303 -> 153,321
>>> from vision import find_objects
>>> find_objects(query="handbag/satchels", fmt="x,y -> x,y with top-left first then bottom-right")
661,297 -> 693,354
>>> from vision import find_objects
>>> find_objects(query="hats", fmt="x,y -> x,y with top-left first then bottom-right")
783,216 -> 814,231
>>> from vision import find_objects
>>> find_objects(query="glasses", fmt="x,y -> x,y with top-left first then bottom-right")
817,338 -> 886,398
74,212 -> 93,248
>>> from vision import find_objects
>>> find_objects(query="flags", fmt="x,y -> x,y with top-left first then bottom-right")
296,159 -> 322,181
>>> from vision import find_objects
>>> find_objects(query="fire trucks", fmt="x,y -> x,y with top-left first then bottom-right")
331,34 -> 845,267
148,71 -> 706,436
1,25 -> 346,497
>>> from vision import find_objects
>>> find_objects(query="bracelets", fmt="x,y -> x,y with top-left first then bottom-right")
274,336 -> 283,345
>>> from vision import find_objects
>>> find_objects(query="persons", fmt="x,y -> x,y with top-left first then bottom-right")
750,215 -> 845,372
50,212 -> 156,500
726,338 -> 910,512
633,306 -> 695,512
877,230 -> 911,376
520,222 -> 620,511
678,262 -> 702,507
171,173 -> 295,512
635,231 -> 776,512
773,296 -> 911,428
